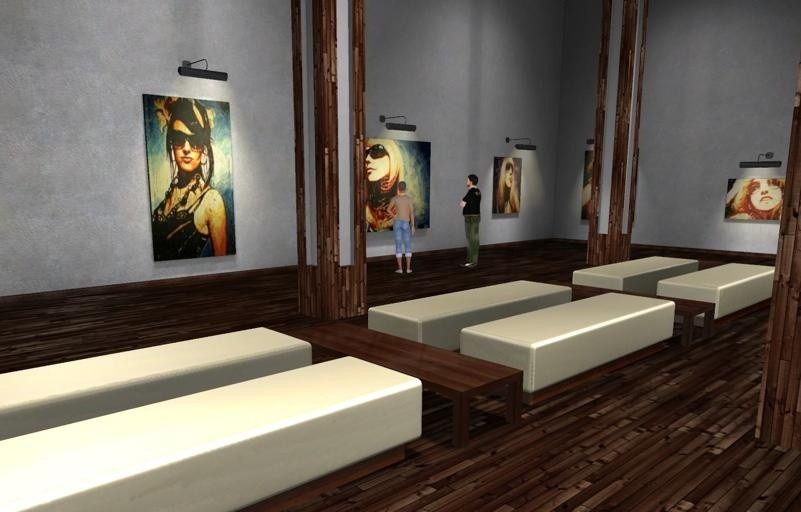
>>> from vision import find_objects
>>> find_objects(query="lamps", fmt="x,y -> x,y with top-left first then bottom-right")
738,152 -> 782,168
506,136 -> 536,150
380,115 -> 417,132
178,57 -> 228,81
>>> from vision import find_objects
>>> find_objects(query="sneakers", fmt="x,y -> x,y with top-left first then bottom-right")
407,270 -> 412,273
395,269 -> 402,274
460,262 -> 477,267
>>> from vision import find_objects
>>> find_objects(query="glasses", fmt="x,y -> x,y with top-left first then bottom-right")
171,131 -> 203,148
366,144 -> 386,158
